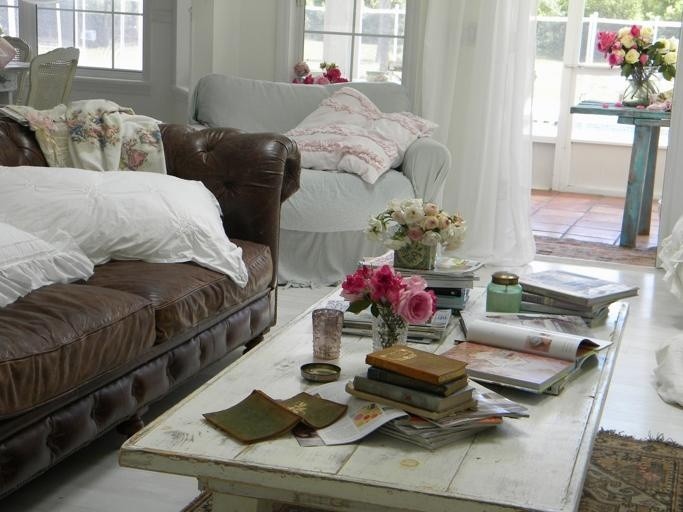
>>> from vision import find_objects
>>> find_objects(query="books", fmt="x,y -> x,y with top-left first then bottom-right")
342,251 -> 485,344
315,344 -> 530,447
517,269 -> 640,328
202,389 -> 347,445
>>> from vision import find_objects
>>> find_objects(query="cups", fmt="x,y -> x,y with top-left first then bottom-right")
311,308 -> 344,358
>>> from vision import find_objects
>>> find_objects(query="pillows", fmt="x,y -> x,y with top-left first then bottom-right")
0,219 -> 92,312
0,162 -> 243,289
282,85 -> 437,184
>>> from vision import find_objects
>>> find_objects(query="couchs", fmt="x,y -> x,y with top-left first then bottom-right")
0,108 -> 301,502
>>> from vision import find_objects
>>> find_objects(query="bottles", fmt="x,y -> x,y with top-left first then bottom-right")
486,272 -> 523,312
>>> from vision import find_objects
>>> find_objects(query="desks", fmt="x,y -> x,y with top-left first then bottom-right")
567,98 -> 669,248
0,62 -> 33,107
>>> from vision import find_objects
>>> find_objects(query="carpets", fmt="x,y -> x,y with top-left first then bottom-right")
179,428 -> 682,512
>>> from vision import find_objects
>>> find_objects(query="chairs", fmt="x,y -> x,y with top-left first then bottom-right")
0,35 -> 31,107
187,72 -> 451,292
15,46 -> 79,110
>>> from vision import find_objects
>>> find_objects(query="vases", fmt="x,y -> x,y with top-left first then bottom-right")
620,67 -> 661,108
392,234 -> 433,271
367,306 -> 410,350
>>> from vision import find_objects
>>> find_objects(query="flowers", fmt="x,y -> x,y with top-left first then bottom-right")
341,259 -> 435,327
596,23 -> 675,85
362,194 -> 464,250
288,59 -> 344,84
